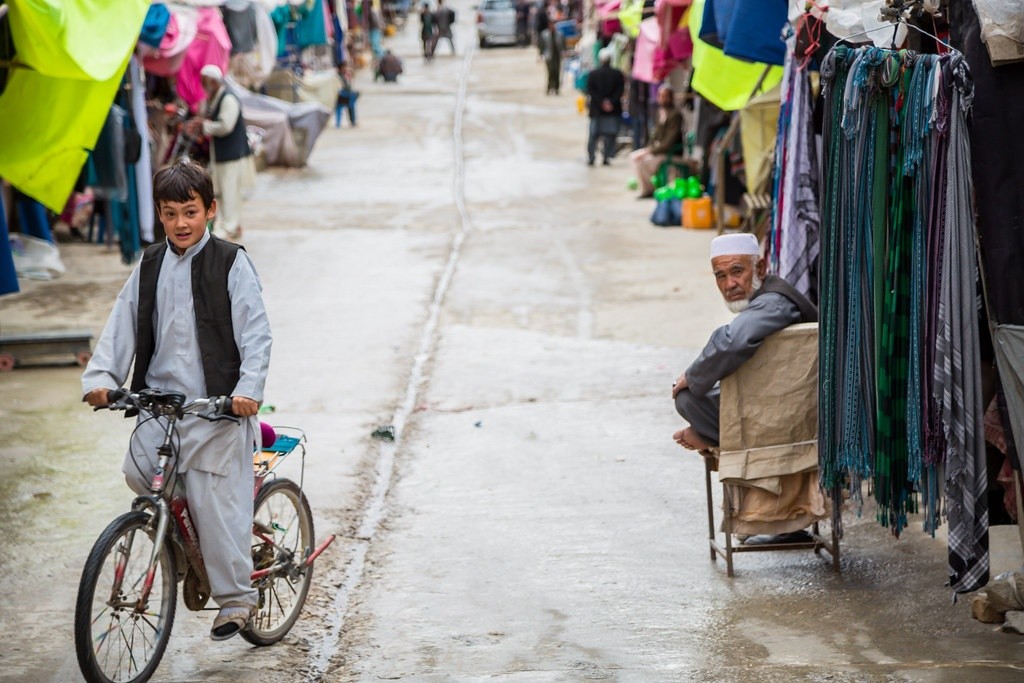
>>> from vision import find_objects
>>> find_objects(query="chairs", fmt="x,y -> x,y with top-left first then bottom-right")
701,323 -> 841,580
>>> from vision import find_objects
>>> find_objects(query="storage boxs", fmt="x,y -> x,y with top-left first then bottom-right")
680,196 -> 710,228
711,204 -> 740,228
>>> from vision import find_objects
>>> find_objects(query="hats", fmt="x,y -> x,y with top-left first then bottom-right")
710,233 -> 761,260
200,64 -> 224,80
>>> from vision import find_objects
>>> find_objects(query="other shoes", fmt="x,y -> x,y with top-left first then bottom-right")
211,225 -> 243,242
744,529 -> 812,544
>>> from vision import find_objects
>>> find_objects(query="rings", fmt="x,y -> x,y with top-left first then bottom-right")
673,381 -> 676,387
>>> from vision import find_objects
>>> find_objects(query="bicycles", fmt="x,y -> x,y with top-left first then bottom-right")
75,391 -> 335,683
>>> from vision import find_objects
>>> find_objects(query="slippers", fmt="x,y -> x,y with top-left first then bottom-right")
183,567 -> 209,611
210,600 -> 257,641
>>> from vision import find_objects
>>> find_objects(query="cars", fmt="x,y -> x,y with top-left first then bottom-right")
471,0 -> 519,48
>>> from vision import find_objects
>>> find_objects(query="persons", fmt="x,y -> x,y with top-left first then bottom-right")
535,0 -> 550,54
540,22 -> 563,95
671,233 -> 819,451
629,83 -> 687,198
363,0 -> 403,82
188,65 -> 253,240
419,0 -> 456,57
513,0 -> 534,47
80,160 -> 273,643
588,47 -> 624,166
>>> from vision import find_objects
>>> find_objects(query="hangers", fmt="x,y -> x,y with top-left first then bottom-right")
833,1 -> 962,56
